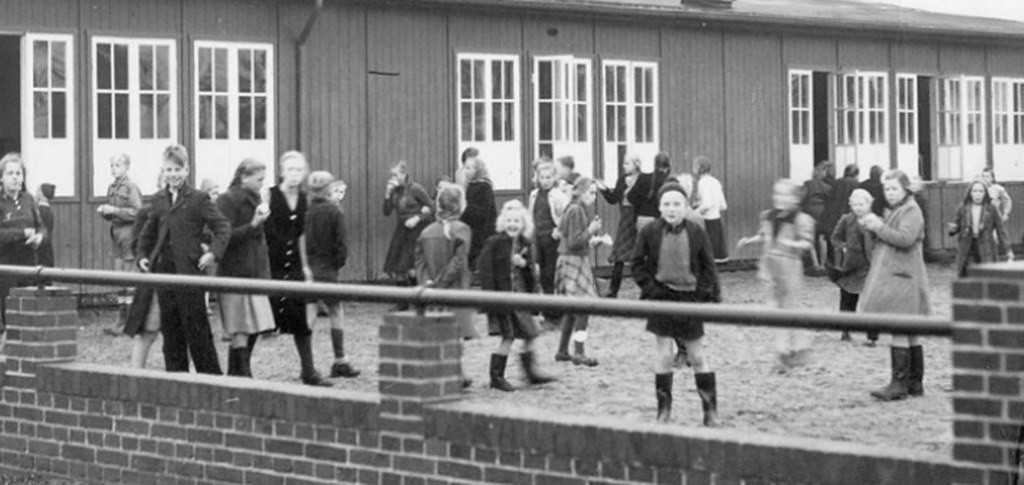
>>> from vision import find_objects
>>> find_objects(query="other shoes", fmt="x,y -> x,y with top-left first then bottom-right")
574,353 -> 597,366
554,350 -> 570,361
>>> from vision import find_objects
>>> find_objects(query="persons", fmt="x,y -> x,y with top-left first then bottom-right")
530,153 -> 581,330
801,163 -> 889,282
414,183 -> 480,386
831,188 -> 879,346
382,160 -> 435,312
631,177 -> 721,425
856,169 -> 934,400
982,167 -> 1012,221
597,151 -> 671,296
738,180 -> 815,365
948,180 -> 1014,279
97,152 -> 143,335
692,156 -> 730,263
131,145 -> 231,374
421,147 -> 498,285
0,154 -> 56,331
478,199 -> 555,392
200,150 -> 360,387
555,177 -> 613,367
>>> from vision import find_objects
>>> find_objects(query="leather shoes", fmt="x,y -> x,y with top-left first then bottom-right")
331,360 -> 362,378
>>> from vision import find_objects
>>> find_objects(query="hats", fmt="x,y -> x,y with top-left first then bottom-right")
657,178 -> 688,200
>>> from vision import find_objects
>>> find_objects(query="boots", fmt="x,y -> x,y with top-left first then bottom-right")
654,372 -> 675,424
224,344 -> 254,381
606,268 -> 622,299
519,349 -> 558,385
909,345 -> 924,396
871,347 -> 910,401
292,334 -> 334,387
488,353 -> 517,391
694,371 -> 723,430
